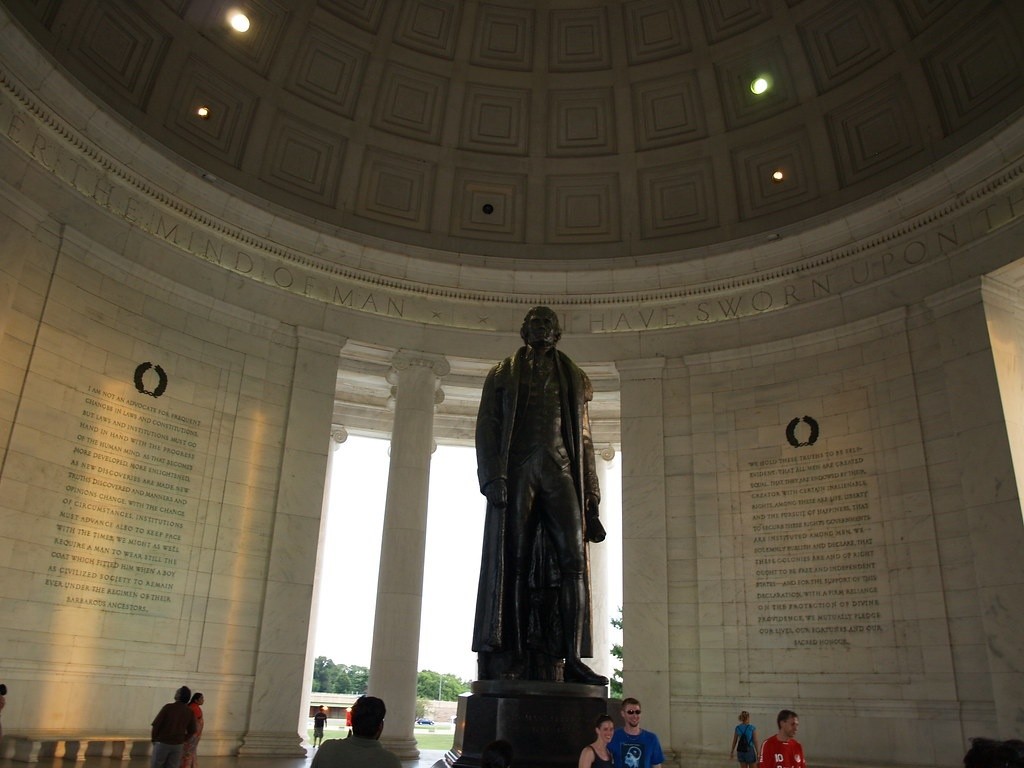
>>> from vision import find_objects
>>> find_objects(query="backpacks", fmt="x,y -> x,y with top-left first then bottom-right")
736,724 -> 753,752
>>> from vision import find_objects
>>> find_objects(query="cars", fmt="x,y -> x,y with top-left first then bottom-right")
417,717 -> 434,725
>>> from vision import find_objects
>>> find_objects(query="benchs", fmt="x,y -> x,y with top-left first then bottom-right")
7,735 -> 153,761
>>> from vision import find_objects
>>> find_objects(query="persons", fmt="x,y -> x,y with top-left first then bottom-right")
0,684 -> 7,759
179,693 -> 204,768
758,710 -> 808,768
606,698 -> 665,768
472,309 -> 606,685
729,711 -> 759,768
150,686 -> 196,768
577,714 -> 615,768
311,695 -> 403,768
480,739 -> 514,768
313,706 -> 327,748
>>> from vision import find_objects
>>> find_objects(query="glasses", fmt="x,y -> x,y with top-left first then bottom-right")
624,709 -> 641,715
